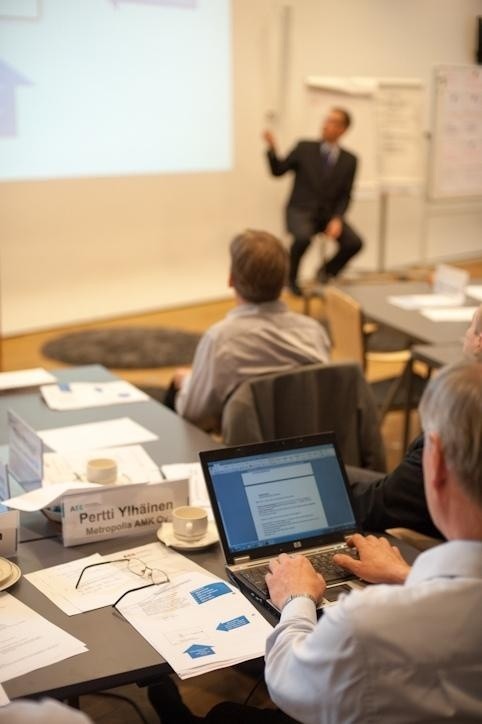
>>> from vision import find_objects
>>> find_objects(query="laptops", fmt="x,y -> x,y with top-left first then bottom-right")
198,430 -> 377,620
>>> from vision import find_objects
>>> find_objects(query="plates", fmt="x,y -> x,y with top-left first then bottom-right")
0,557 -> 12,581
0,561 -> 22,591
156,524 -> 220,553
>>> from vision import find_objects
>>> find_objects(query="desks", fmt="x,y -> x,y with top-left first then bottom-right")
0,534 -> 423,710
0,361 -> 440,542
401,344 -> 466,457
334,279 -> 480,342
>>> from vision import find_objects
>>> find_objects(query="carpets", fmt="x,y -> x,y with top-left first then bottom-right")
41,324 -> 207,370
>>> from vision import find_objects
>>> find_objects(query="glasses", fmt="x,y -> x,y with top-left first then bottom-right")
77,556 -> 170,609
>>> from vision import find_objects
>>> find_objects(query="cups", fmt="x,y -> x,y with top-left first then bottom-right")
86,458 -> 118,484
172,506 -> 208,538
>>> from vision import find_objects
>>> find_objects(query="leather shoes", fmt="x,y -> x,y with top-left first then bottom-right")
287,278 -> 303,295
146,677 -> 194,723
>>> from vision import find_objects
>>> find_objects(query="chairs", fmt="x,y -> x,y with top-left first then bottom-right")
326,289 -> 436,440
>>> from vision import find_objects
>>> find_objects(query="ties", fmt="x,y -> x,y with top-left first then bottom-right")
322,149 -> 331,171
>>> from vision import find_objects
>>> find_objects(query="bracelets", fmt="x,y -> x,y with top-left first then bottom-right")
281,592 -> 317,609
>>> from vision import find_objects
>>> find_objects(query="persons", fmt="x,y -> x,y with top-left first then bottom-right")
206,355 -> 482,722
167,228 -> 334,445
263,109 -> 365,298
346,305 -> 482,540
